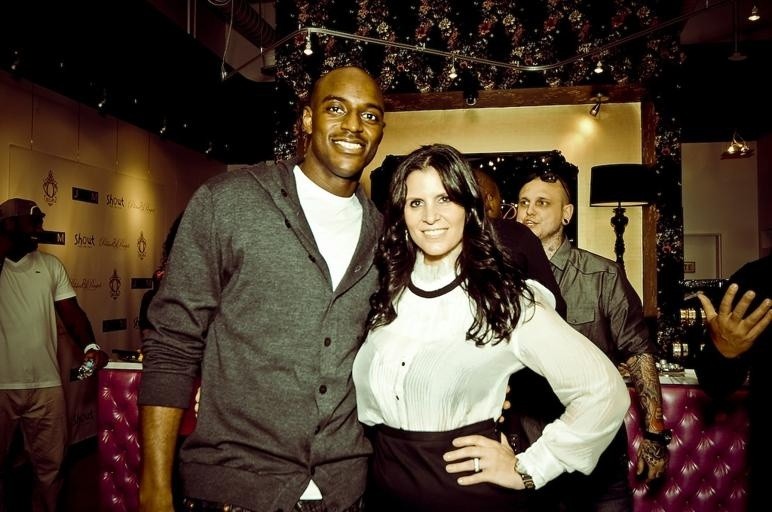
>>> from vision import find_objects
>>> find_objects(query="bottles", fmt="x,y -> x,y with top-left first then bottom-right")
76,358 -> 96,381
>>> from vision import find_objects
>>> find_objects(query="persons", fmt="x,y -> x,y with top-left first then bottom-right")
130,66 -> 413,511
1,198 -> 111,511
473,167 -> 568,511
138,211 -> 209,377
351,144 -> 631,510
514,170 -> 673,512
695,255 -> 772,511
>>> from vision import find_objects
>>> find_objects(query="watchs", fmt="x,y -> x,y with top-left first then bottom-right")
514,459 -> 535,491
643,430 -> 672,445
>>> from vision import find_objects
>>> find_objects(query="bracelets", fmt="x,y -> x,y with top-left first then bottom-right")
83,343 -> 100,354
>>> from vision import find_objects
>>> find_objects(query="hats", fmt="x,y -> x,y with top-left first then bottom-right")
0,198 -> 46,221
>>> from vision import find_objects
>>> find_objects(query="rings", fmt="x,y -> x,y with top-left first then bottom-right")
474,458 -> 480,472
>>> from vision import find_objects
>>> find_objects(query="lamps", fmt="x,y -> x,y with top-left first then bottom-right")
727,0 -> 747,62
455,59 -> 479,105
590,89 -> 607,117
260,47 -> 277,76
747,0 -> 762,22
589,164 -> 655,281
594,53 -> 606,74
720,127 -> 755,159
303,28 -> 313,56
